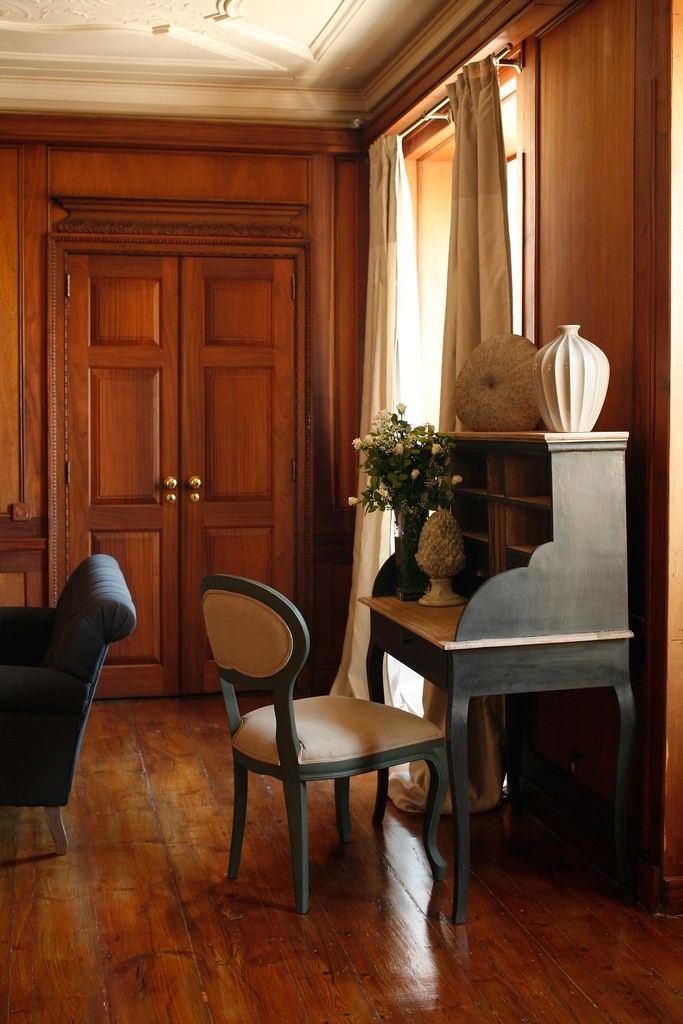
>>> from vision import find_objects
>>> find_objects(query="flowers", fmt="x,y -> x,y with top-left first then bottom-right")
348,399 -> 464,508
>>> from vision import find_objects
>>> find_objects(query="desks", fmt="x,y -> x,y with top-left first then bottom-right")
354,431 -> 639,925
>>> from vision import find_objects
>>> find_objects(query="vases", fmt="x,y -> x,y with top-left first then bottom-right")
530,324 -> 612,433
391,503 -> 432,602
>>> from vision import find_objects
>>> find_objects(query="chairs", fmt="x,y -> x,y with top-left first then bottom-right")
198,574 -> 451,914
0,552 -> 138,854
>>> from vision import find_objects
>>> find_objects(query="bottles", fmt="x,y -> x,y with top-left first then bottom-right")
529,324 -> 611,433
